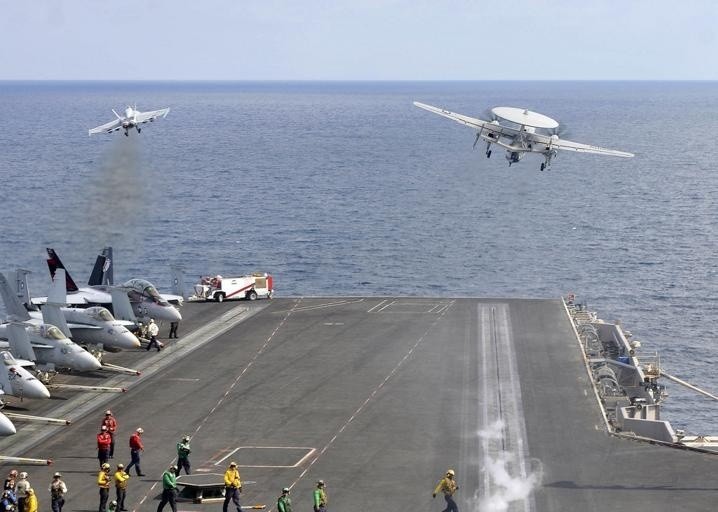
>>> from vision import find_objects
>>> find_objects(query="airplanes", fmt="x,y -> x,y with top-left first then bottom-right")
88,102 -> 171,139
0,246 -> 185,439
412,100 -> 635,171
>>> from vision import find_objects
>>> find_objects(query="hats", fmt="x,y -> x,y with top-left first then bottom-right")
150,318 -> 154,323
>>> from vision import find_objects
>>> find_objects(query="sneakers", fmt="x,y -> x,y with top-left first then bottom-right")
124,470 -> 130,477
137,472 -> 147,476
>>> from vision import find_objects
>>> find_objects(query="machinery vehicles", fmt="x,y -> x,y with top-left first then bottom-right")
188,273 -> 272,302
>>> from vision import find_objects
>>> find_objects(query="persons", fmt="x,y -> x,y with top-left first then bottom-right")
434,469 -> 460,512
169,307 -> 187,338
207,277 -> 219,290
145,319 -> 161,352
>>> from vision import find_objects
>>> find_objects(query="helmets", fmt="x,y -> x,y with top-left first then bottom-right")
9,469 -> 18,478
101,462 -> 111,470
316,479 -> 327,488
229,461 -> 238,468
136,427 -> 145,435
117,463 -> 125,469
105,410 -> 113,416
19,471 -> 29,479
52,471 -> 63,478
100,425 -> 108,432
182,435 -> 190,442
281,487 -> 291,495
445,468 -> 456,477
170,464 -> 179,471
